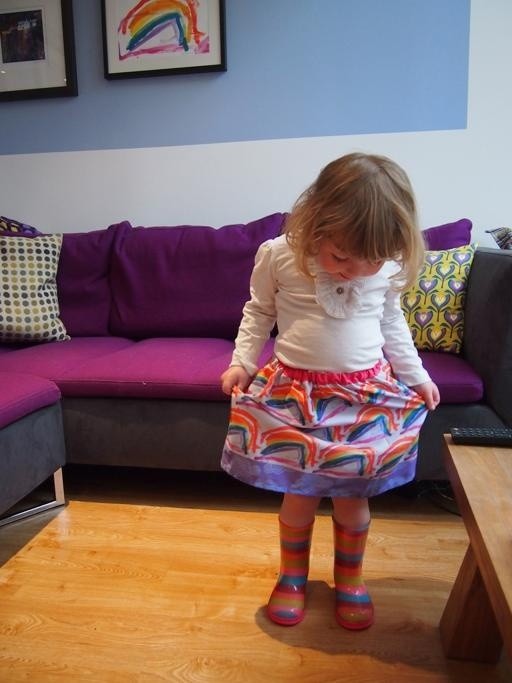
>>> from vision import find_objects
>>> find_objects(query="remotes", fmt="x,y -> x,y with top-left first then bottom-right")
450,428 -> 512,448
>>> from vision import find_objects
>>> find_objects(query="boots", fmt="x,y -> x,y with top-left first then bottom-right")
331,514 -> 374,633
266,511 -> 316,628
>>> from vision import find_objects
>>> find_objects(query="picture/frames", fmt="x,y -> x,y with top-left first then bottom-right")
1,0 -> 226,103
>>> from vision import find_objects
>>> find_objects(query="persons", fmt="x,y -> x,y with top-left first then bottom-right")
218,148 -> 443,634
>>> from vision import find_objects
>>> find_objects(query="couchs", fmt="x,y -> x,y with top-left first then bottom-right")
1,216 -> 511,536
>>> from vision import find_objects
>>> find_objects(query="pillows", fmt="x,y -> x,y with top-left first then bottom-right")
398,243 -> 478,352
0,231 -> 71,341
